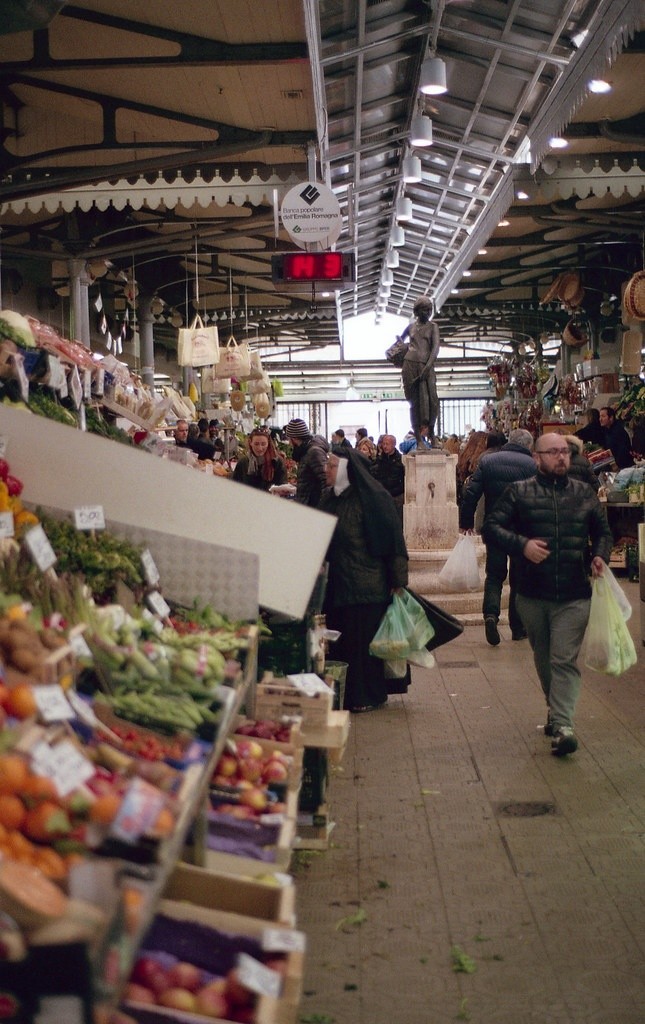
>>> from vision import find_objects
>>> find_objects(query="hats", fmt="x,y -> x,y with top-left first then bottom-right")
286,418 -> 311,438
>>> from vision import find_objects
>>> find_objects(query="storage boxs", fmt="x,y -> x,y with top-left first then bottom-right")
119,671 -> 349,1024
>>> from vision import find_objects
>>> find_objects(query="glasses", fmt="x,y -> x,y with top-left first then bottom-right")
538,447 -> 572,458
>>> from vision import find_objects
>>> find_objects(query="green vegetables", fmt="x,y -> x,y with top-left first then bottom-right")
277,441 -> 294,460
3,394 -> 151,452
35,504 -> 141,595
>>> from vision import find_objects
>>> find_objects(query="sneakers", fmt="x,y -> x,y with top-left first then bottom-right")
551,726 -> 578,759
544,708 -> 554,737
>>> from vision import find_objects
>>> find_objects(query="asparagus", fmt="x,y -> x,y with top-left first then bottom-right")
0,542 -> 91,627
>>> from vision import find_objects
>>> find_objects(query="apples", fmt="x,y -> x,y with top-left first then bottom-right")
212,718 -> 289,819
125,956 -> 287,1024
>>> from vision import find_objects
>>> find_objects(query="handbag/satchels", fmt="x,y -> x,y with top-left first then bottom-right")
215,334 -> 250,378
248,371 -> 272,393
201,364 -> 231,394
575,573 -> 638,675
438,530 -> 481,592
241,380 -> 248,394
368,585 -> 464,680
589,558 -> 632,622
178,314 -> 220,369
237,350 -> 264,383
271,378 -> 284,398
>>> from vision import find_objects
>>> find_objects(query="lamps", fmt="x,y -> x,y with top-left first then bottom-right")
376,33 -> 448,327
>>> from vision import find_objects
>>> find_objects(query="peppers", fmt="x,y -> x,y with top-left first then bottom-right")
0,457 -> 38,530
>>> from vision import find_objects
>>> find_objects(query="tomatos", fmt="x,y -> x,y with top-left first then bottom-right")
167,620 -> 199,638
96,726 -> 178,761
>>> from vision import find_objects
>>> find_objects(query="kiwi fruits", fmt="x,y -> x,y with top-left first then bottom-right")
0,616 -> 71,677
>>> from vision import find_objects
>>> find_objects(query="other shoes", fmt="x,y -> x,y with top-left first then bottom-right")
511,630 -> 529,641
345,694 -> 389,714
485,617 -> 501,646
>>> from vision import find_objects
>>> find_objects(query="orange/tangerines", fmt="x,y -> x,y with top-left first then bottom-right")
1,686 -> 171,924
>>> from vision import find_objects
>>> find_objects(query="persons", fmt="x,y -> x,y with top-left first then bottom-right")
232,428 -> 287,494
458,428 -> 538,645
562,435 -> 601,494
284,417 -> 331,508
397,294 -> 442,451
572,406 -> 636,470
441,429 -> 506,535
329,428 -> 405,523
316,445 -> 410,714
399,420 -> 431,455
173,418 -> 225,461
481,433 -> 611,755
